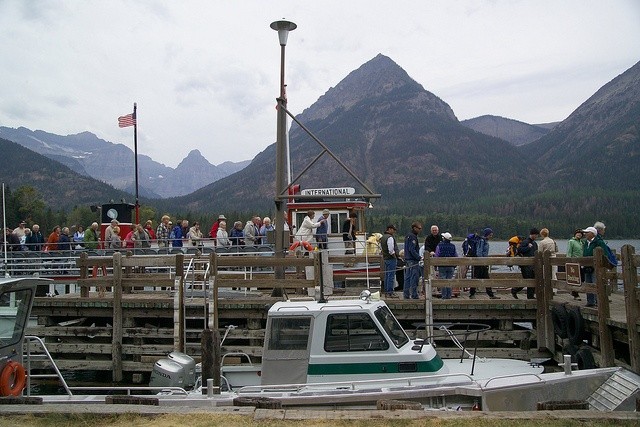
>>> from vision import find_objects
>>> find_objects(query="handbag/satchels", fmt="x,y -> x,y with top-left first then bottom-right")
596,238 -> 617,269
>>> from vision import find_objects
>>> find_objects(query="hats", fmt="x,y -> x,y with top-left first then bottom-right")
19,220 -> 27,224
307,210 -> 315,217
575,229 -> 583,235
387,225 -> 398,231
581,226 -> 598,236
441,232 -> 452,240
484,227 -> 493,238
322,209 -> 330,215
218,215 -> 226,220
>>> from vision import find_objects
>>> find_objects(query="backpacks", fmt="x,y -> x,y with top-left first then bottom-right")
461,233 -> 480,257
506,235 -> 530,271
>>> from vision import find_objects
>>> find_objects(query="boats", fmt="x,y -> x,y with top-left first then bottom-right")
150,250 -> 544,393
89,84 -> 383,273
0,278 -> 616,410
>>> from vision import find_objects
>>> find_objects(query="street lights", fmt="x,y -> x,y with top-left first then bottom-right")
270,17 -> 296,296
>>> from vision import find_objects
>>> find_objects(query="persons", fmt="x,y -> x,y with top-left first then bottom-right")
143,219 -> 155,242
295,210 -> 324,241
6,221 -> 44,251
210,215 -> 227,248
168,221 -> 172,239
217,221 -> 232,246
381,224 -> 400,298
425,225 -> 441,257
170,220 -> 183,247
189,222 -> 203,246
156,215 -> 170,247
434,233 -> 458,299
594,222 -> 606,239
565,226 -> 585,301
284,211 -> 289,231
582,226 -> 607,307
511,228 -> 538,300
73,225 -> 85,249
229,221 -> 243,245
48,225 -> 70,250
245,217 -> 261,245
84,222 -> 99,249
123,225 -> 136,248
343,212 -> 357,254
316,209 -> 330,249
258,217 -> 276,244
469,228 -> 501,299
538,228 -> 558,295
403,222 -> 424,299
131,224 -> 151,248
104,220 -> 121,249
182,219 -> 190,239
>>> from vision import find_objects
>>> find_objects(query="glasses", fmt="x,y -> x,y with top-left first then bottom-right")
418,227 -> 421,230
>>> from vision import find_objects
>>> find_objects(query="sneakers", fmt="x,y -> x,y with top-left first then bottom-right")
469,293 -> 476,298
386,294 -> 399,297
511,290 -> 518,298
489,295 -> 501,299
527,296 -> 536,299
